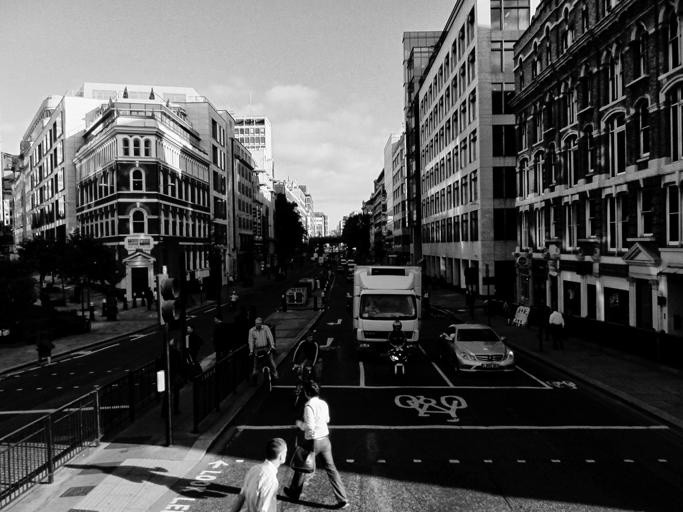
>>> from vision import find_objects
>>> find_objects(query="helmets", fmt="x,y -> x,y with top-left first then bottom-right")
393,320 -> 402,330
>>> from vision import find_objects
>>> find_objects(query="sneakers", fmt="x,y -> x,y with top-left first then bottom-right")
334,501 -> 349,511
284,486 -> 300,501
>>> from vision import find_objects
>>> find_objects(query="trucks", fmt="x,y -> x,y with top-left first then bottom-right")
344,264 -> 430,351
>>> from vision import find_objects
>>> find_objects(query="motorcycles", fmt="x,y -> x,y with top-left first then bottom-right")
385,342 -> 413,376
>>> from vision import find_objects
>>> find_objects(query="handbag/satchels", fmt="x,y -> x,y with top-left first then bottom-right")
290,446 -> 316,474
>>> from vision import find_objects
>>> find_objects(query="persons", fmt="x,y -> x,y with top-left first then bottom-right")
548,307 -> 565,351
282,378 -> 349,508
247,315 -> 278,387
290,332 -> 322,388
386,321 -> 406,364
230,437 -> 287,512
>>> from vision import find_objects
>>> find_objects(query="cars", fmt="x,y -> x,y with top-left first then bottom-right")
436,323 -> 517,375
308,252 -> 357,281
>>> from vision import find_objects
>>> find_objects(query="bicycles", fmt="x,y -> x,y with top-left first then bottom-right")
252,346 -> 276,393
289,363 -> 312,416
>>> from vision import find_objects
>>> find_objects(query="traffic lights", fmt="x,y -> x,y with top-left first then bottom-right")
155,272 -> 183,327
